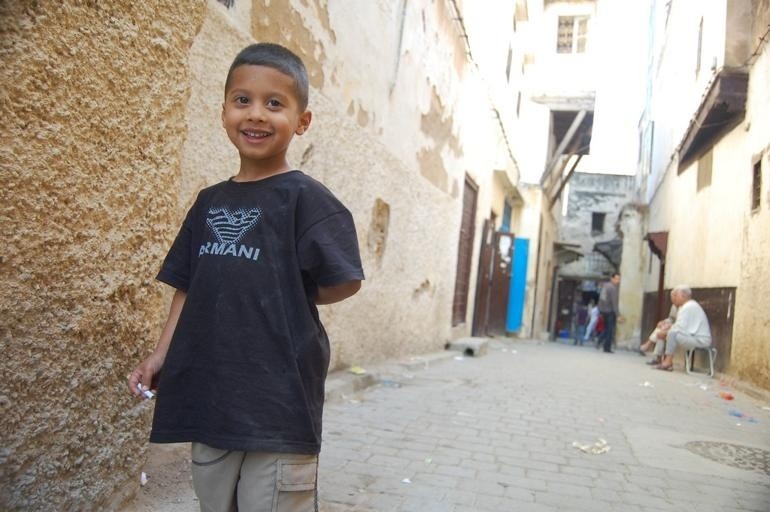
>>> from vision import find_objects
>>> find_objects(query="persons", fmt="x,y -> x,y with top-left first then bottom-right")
631,287 -> 679,366
655,283 -> 713,372
594,272 -> 626,353
128,42 -> 366,510
568,297 -> 605,349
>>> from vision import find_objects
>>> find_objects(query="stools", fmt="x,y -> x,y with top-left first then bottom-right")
685,346 -> 718,378
664,340 -> 694,371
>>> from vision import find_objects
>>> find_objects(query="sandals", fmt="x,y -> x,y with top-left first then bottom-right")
632,346 -> 673,372
